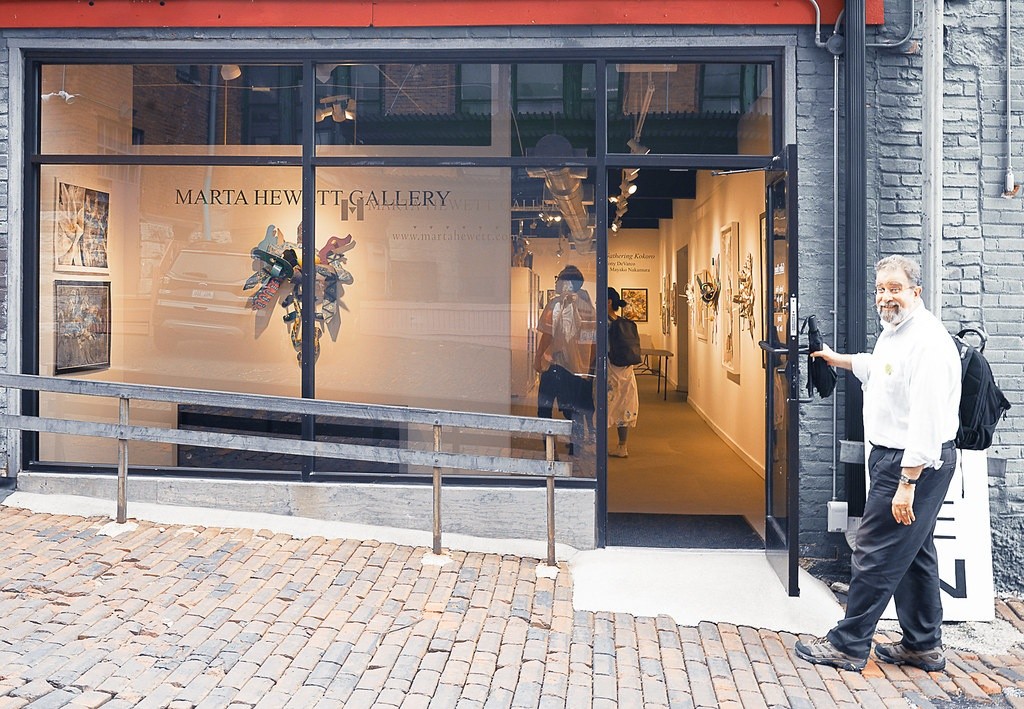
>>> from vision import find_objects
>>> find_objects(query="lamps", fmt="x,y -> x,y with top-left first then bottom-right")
612,140 -> 650,233
530,222 -> 537,229
221,65 -> 241,81
316,64 -> 337,83
316,96 -> 355,124
556,248 -> 563,256
41,91 -> 75,104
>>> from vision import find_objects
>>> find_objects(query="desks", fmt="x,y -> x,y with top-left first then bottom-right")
633,348 -> 674,401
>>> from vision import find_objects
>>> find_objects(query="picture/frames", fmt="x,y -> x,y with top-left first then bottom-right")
547,290 -> 555,307
620,288 -> 649,323
51,280 -> 111,375
51,176 -> 112,276
692,270 -> 710,342
722,222 -> 743,375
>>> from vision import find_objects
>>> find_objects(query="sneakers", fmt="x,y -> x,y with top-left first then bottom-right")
795,636 -> 867,671
875,641 -> 946,672
608,445 -> 628,458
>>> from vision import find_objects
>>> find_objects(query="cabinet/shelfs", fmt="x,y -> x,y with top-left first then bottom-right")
510,266 -> 540,397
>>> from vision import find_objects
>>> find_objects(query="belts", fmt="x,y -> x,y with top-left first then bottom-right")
943,441 -> 954,449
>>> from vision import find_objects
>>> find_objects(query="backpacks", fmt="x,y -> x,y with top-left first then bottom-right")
608,315 -> 643,366
948,328 -> 1011,500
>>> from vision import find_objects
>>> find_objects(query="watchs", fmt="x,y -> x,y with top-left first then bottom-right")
898,473 -> 919,486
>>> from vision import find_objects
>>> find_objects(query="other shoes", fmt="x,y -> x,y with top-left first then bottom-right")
544,450 -> 559,461
586,438 -> 596,445
567,444 -> 581,457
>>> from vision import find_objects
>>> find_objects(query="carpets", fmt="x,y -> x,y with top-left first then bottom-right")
607,512 -> 766,555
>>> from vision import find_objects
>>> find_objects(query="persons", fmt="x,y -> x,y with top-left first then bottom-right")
535,264 -> 595,473
558,289 -> 598,445
588,286 -> 639,457
795,257 -> 962,672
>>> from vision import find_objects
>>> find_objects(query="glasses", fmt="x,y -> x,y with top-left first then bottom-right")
875,281 -> 915,294
555,275 -> 560,282
